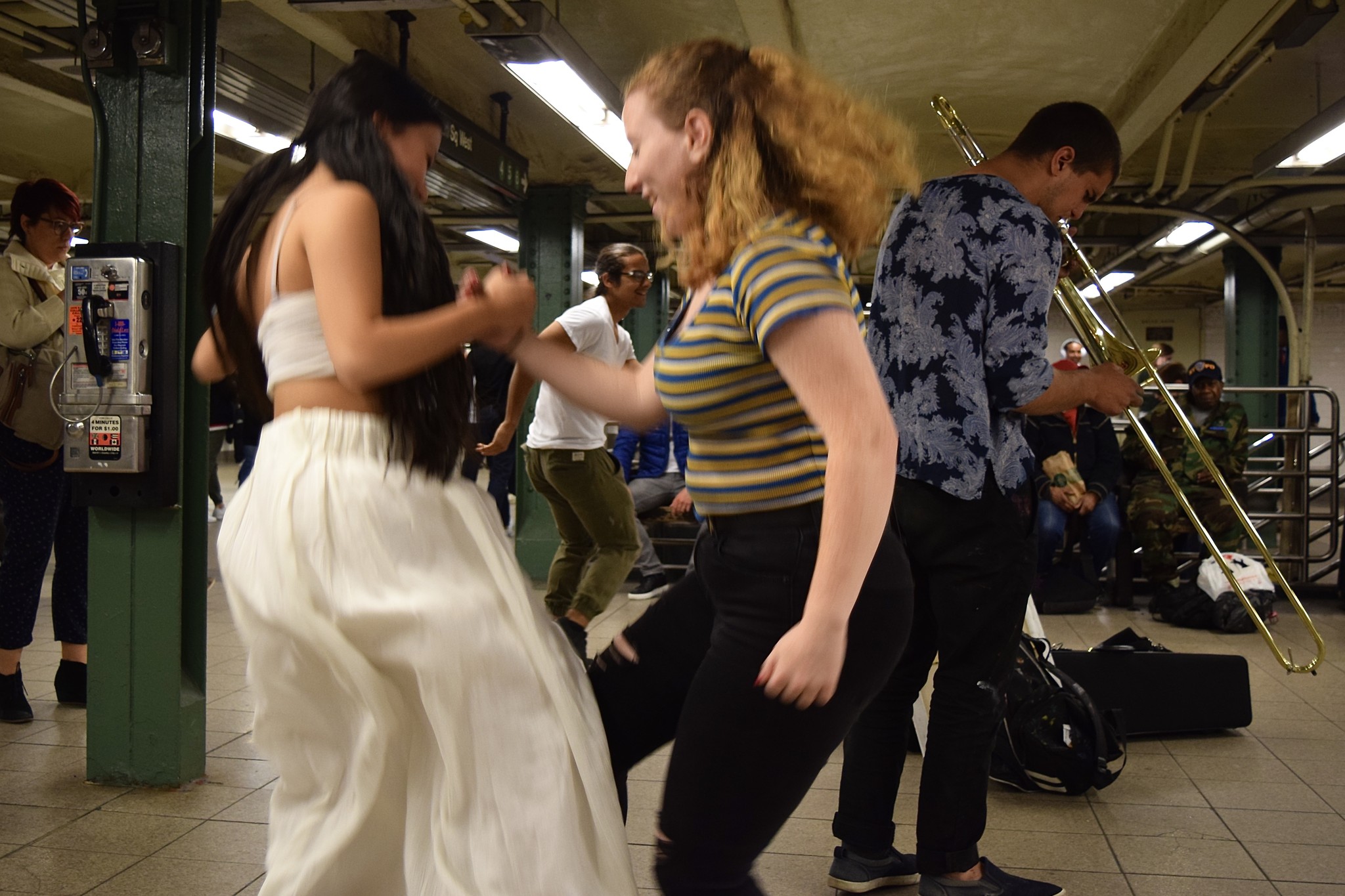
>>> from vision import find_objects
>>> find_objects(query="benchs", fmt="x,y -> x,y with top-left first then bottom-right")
641,505 -> 1245,603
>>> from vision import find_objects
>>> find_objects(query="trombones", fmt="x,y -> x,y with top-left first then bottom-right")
929,93 -> 1326,676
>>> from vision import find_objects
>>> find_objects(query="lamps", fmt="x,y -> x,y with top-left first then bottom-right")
1252,96 -> 1345,180
214,47 -> 522,254
1146,196 -> 1237,251
1081,259 -> 1137,300
458,1 -> 632,171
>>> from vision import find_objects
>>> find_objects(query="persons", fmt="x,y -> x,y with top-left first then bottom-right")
1123,360 -> 1250,605
0,177 -> 88,716
475,241 -> 652,669
189,56 -> 640,896
1024,361 -> 1122,610
612,417 -> 707,599
460,38 -> 922,896
1060,337 -> 1188,416
827,99 -> 1144,895
462,340 -> 515,538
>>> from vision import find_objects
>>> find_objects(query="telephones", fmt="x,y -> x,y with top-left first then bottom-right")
58,256 -> 153,404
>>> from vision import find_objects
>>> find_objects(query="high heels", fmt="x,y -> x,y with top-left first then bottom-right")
0,661 -> 35,722
53,658 -> 88,707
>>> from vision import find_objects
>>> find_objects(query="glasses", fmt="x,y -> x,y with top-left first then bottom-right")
36,217 -> 84,236
615,270 -> 653,282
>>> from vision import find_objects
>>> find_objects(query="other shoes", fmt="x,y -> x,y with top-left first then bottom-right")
213,506 -> 226,519
827,844 -> 921,893
920,854 -> 1065,896
1148,591 -> 1167,621
555,616 -> 588,672
1094,579 -> 1114,607
628,570 -> 671,600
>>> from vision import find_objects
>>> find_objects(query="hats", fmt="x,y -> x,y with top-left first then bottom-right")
1051,358 -> 1089,372
1059,337 -> 1087,357
1188,359 -> 1223,384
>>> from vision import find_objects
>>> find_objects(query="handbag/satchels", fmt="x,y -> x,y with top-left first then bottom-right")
1168,552 -> 1275,634
987,629 -> 1127,795
0,277 -> 65,449
1040,542 -> 1099,615
